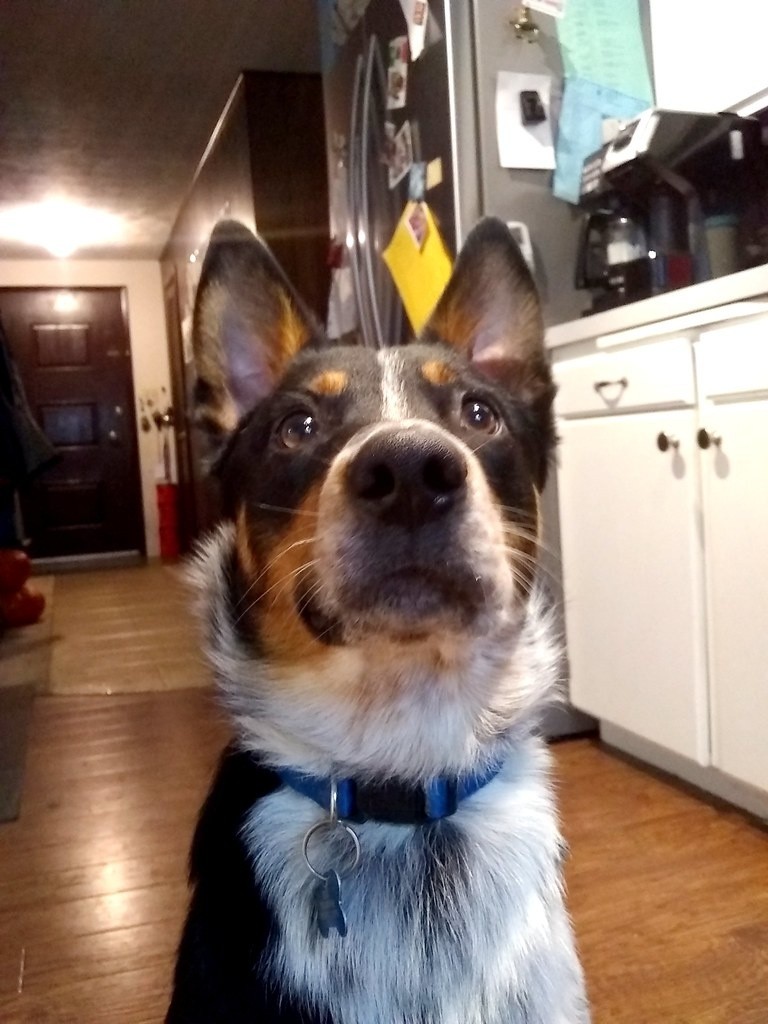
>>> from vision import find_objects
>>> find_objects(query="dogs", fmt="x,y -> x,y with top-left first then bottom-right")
162,217 -> 592,1024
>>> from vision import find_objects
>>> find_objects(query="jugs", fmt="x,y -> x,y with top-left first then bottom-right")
570,204 -> 648,289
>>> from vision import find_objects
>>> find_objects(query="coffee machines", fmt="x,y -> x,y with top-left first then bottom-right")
574,106 -> 763,319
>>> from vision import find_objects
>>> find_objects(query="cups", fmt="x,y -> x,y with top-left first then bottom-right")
704,214 -> 740,278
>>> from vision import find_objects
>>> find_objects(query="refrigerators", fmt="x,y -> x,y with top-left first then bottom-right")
323,0 -> 659,347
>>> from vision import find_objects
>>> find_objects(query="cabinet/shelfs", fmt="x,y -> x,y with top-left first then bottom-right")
543,260 -> 768,820
